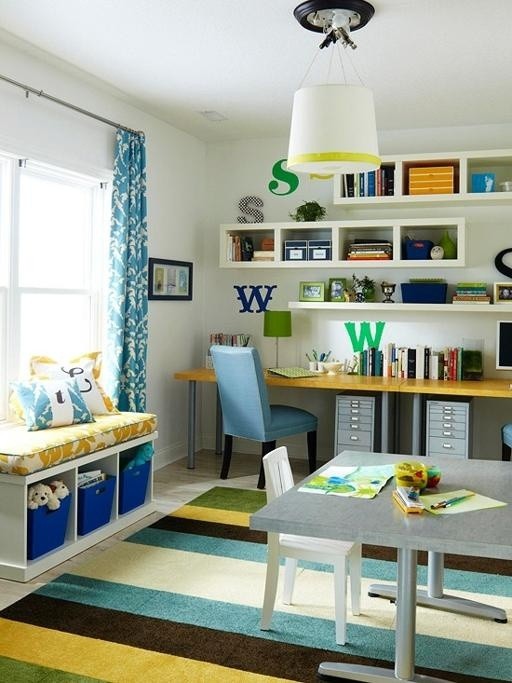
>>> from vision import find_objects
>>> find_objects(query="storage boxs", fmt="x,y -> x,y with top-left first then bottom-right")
401,282 -> 447,304
284,239 -> 332,260
405,239 -> 434,259
408,166 -> 454,194
28,458 -> 150,560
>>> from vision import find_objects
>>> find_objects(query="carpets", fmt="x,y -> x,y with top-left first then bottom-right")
0,487 -> 512,683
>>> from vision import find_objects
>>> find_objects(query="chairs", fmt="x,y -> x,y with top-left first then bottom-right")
501,424 -> 512,461
208,345 -> 317,489
171,368 -> 512,468
259,446 -> 361,645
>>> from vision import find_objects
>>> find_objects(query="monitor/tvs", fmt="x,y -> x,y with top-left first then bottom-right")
497,320 -> 512,370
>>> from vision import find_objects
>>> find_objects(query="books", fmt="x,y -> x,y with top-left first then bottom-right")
356,343 -> 463,381
347,238 -> 393,260
452,281 -> 490,306
343,166 -> 395,197
209,334 -> 248,355
266,368 -> 319,380
225,232 -> 274,263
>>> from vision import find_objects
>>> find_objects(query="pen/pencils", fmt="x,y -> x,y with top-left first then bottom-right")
430,494 -> 475,509
306,349 -> 331,362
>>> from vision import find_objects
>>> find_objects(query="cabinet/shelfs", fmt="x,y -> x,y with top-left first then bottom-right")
331,149 -> 511,208
0,410 -> 160,584
218,217 -> 464,269
333,391 -> 381,458
426,394 -> 474,460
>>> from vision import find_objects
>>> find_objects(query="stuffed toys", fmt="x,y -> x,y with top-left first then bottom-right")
27,483 -> 61,511
49,479 -> 70,500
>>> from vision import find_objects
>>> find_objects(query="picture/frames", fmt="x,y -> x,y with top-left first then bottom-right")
329,278 -> 347,302
148,258 -> 193,301
300,281 -> 325,302
493,282 -> 512,305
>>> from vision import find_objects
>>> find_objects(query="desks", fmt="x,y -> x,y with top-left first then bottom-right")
249,450 -> 512,683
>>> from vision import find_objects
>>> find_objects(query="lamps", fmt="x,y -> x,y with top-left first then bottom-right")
263,310 -> 291,368
285,1 -> 380,175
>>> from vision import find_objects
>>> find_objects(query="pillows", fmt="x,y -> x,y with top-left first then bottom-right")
1,351 -> 122,432
